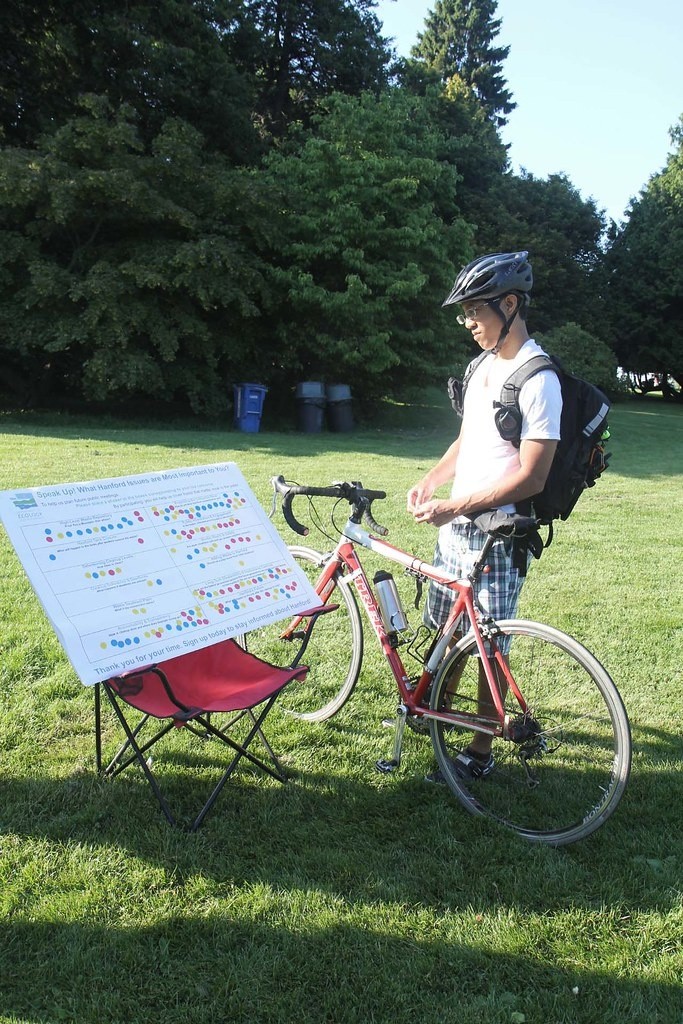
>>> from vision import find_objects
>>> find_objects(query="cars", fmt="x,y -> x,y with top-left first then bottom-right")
640,379 -> 674,394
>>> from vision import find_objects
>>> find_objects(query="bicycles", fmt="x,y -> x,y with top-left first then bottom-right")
231,474 -> 633,851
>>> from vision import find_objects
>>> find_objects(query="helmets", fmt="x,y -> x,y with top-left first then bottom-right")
441,251 -> 533,309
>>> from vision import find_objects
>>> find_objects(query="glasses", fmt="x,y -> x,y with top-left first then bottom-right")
455,292 -> 508,326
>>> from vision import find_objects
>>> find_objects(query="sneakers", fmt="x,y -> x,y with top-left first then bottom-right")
423,749 -> 496,787
384,712 -> 456,736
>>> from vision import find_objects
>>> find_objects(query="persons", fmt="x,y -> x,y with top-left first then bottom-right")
382,251 -> 564,788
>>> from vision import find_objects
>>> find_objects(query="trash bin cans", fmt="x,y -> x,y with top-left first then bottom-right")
295,381 -> 327,433
233,382 -> 269,435
328,383 -> 355,431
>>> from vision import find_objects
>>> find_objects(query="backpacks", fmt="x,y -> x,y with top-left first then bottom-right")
458,347 -> 609,522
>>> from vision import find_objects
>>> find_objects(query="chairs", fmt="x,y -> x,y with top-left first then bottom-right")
95,604 -> 340,834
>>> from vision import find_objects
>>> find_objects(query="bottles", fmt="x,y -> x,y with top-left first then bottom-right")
373,570 -> 408,635
